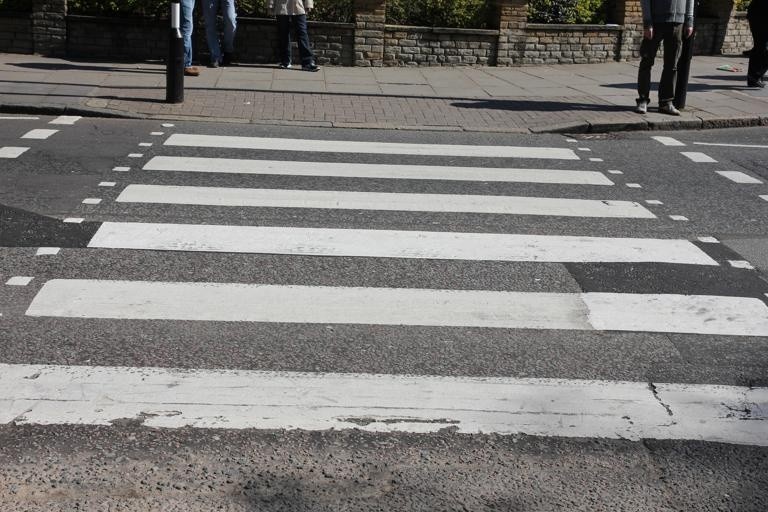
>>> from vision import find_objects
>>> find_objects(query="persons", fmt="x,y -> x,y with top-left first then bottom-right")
267,1 -> 320,73
635,1 -> 695,116
200,1 -> 240,67
179,1 -> 200,75
744,1 -> 768,90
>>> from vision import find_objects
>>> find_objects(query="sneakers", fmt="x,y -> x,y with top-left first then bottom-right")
634,99 -> 647,114
658,101 -> 681,115
302,63 -> 320,72
184,67 -> 200,76
